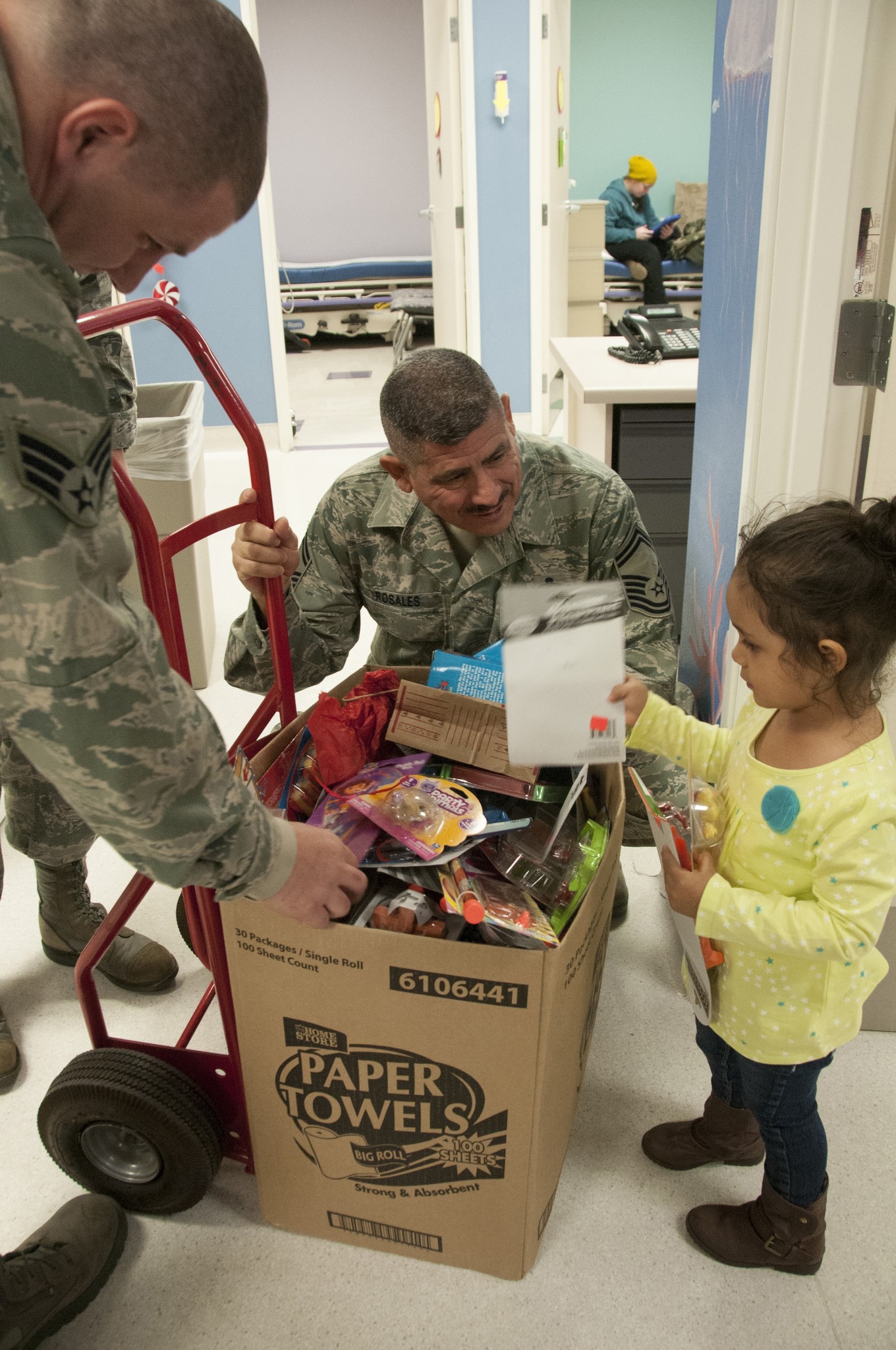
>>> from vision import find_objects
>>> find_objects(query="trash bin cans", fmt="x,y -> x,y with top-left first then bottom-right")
118,381 -> 216,689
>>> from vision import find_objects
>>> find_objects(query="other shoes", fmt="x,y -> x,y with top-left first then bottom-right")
608,864 -> 629,932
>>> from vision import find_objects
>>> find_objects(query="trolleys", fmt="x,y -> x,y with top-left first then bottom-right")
36,301 -> 299,1218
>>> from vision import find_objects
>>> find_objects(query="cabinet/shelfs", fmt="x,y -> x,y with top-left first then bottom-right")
548,336 -> 699,642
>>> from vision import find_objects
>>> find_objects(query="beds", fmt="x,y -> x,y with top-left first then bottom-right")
275,256 -> 433,338
604,249 -> 703,327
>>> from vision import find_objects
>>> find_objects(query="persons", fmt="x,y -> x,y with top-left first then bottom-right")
598,155 -> 682,305
223,349 -> 680,929
0,0 -> 368,1350
609,495 -> 894,1275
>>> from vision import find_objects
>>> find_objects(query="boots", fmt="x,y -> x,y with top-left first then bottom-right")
641,1090 -> 767,1171
686,1171 -> 832,1274
0,1008 -> 22,1095
31,854 -> 178,991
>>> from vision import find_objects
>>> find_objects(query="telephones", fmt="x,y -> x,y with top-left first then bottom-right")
608,304 -> 700,364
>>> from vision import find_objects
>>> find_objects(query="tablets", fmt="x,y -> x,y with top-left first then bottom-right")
652,215 -> 681,234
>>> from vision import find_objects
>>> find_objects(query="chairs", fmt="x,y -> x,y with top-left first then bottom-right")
393,310 -> 434,366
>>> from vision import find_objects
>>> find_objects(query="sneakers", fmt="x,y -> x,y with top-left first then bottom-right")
0,1194 -> 129,1350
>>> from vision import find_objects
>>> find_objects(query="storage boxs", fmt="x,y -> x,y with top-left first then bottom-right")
220,665 -> 625,1280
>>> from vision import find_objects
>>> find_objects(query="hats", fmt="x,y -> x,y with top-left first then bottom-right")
626,158 -> 657,182
